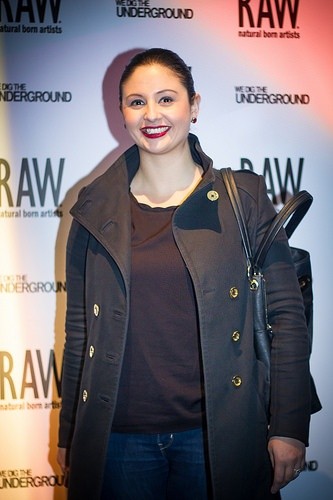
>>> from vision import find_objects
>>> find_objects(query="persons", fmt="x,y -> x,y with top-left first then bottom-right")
55,47 -> 322,500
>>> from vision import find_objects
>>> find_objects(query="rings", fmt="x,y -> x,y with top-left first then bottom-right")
291,468 -> 301,475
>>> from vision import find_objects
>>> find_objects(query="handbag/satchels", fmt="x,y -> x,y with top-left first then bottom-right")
221,167 -> 323,426
